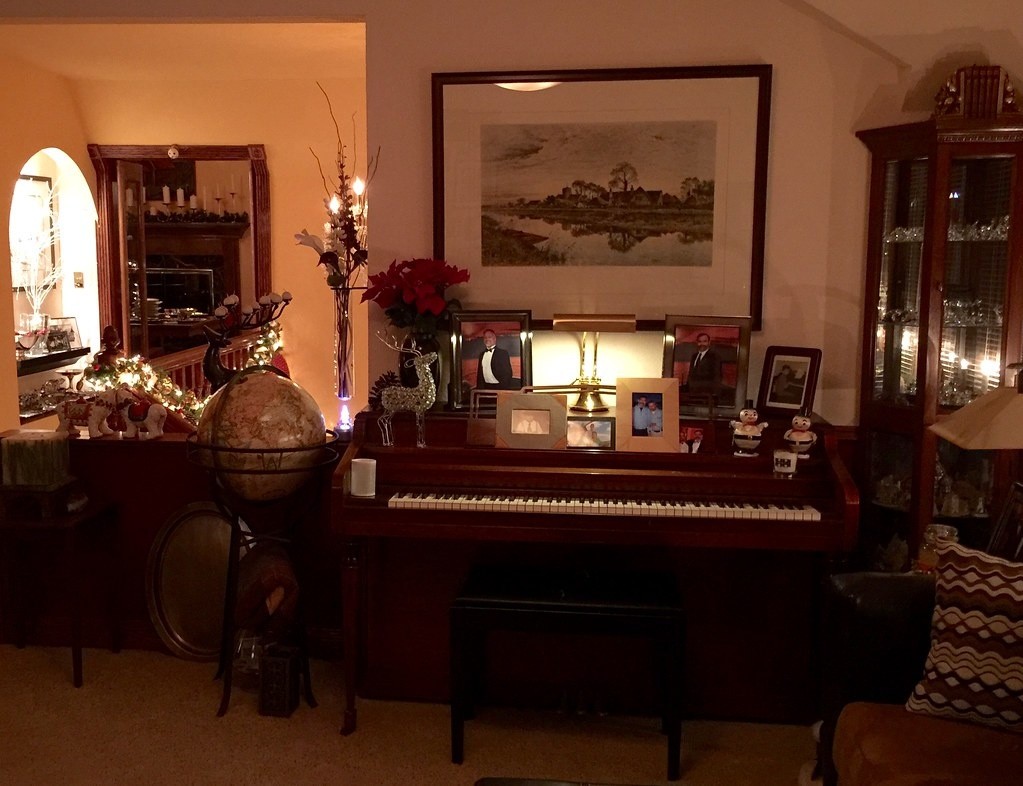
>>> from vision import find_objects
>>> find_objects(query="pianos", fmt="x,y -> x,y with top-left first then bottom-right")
322,398 -> 864,737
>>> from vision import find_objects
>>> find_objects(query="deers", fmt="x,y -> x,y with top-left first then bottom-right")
375,328 -> 438,447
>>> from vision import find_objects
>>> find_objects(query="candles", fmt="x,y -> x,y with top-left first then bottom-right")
126,172 -> 236,209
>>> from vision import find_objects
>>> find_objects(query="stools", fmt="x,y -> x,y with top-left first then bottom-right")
448,554 -> 686,780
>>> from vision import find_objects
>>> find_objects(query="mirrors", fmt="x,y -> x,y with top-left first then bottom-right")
86,142 -> 273,394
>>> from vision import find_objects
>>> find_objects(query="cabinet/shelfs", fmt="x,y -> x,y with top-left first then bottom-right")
854,114 -> 1023,572
17,347 -> 90,422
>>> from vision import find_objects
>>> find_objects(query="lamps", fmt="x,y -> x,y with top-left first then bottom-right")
926,363 -> 1023,450
552,313 -> 637,412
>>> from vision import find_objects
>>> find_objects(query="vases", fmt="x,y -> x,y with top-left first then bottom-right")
398,332 -> 441,392
332,287 -> 355,401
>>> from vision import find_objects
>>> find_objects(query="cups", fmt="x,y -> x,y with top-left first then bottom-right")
50,316 -> 82,349
350,458 -> 376,496
869,214 -> 1010,514
773,444 -> 799,478
18,314 -> 47,357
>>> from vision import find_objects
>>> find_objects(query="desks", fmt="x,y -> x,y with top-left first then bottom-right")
127,315 -> 215,338
0,499 -> 122,689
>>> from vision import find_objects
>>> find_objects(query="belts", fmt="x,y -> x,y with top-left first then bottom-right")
649,430 -> 663,432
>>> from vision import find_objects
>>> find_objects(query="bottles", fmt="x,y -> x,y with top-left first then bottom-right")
917,524 -> 959,573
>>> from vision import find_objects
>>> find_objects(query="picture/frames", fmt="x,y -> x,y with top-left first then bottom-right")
10,175 -> 57,291
756,346 -> 822,417
433,64 -> 773,331
50,317 -> 82,347
986,481 -> 1023,559
446,310 -> 532,414
496,377 -> 680,454
661,314 -> 752,419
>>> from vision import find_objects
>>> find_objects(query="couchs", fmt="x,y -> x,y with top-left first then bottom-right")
798,570 -> 1023,786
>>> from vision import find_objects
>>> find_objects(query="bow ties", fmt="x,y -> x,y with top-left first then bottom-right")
694,440 -> 699,443
485,346 -> 496,352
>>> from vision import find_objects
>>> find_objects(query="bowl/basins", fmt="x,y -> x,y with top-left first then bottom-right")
135,298 -> 197,320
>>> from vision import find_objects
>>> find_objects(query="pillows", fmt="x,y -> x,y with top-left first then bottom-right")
905,537 -> 1023,735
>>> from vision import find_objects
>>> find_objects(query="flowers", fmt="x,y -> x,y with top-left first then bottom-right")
291,80 -> 383,397
360,258 -> 470,341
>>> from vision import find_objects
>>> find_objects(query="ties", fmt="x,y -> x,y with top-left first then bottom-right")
527,422 -> 532,432
640,407 -> 643,412
696,353 -> 702,367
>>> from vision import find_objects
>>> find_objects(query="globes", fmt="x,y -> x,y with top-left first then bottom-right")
197,373 -> 324,503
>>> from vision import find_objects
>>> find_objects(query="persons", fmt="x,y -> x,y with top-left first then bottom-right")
774,365 -> 797,404
583,421 -> 600,447
633,395 -> 651,437
476,329 -> 512,389
686,332 -> 722,403
648,400 -> 662,437
516,410 -> 543,434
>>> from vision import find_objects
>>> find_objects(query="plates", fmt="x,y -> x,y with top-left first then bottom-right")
129,311 -> 208,323
